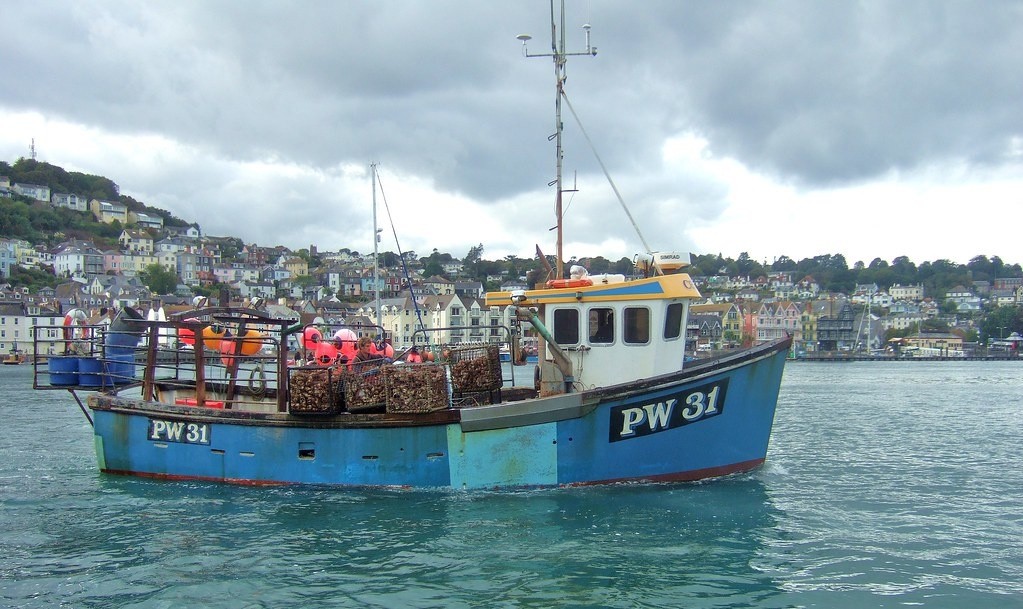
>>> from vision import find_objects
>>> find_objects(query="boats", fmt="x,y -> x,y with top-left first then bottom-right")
32,2 -> 797,492
2,349 -> 22,364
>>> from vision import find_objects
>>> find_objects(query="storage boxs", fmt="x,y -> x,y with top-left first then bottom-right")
286,343 -> 503,416
175,397 -> 224,409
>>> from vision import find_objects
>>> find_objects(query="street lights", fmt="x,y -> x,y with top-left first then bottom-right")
996,326 -> 1008,345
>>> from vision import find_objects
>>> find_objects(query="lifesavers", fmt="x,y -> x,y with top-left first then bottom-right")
546,277 -> 593,289
63,310 -> 90,348
